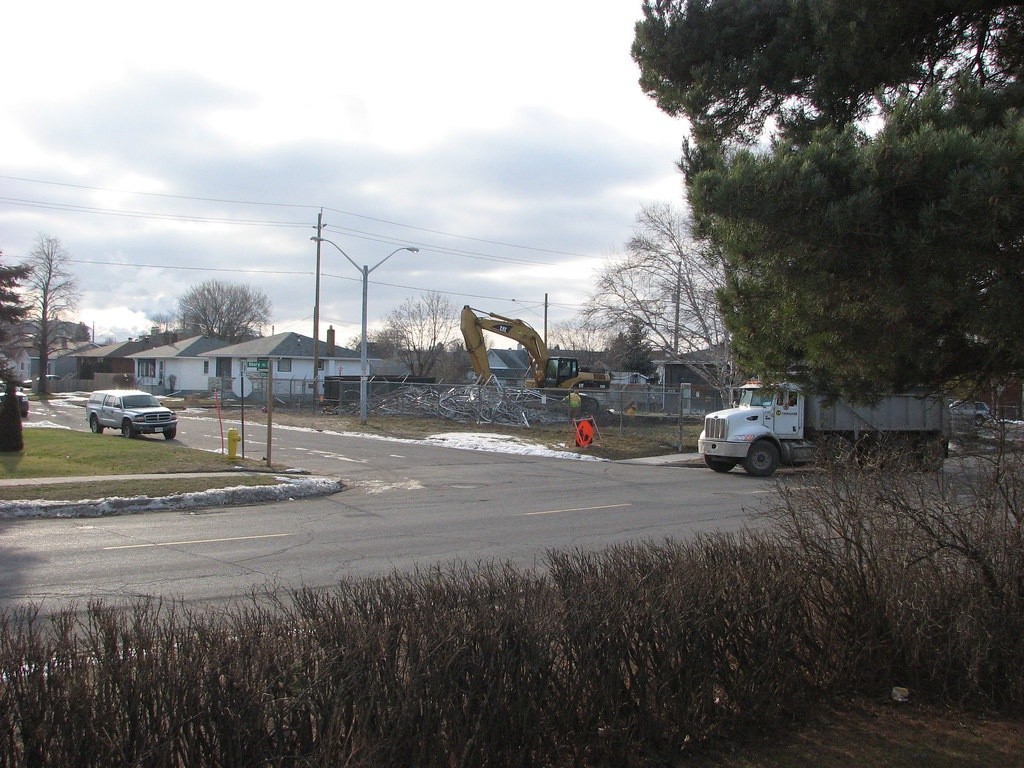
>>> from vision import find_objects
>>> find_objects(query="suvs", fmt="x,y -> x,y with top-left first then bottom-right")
949,400 -> 992,426
0,381 -> 30,417
85,388 -> 178,440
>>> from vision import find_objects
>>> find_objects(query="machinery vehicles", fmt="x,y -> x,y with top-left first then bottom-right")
459,305 -> 614,414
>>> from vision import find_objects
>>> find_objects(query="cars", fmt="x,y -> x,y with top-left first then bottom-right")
22,374 -> 62,388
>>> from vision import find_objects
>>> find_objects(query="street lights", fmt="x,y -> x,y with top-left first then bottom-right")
308,236 -> 420,425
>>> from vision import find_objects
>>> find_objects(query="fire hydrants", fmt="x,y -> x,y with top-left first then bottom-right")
226,427 -> 242,460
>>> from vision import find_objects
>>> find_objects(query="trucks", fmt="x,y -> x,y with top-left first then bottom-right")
698,371 -> 951,477
